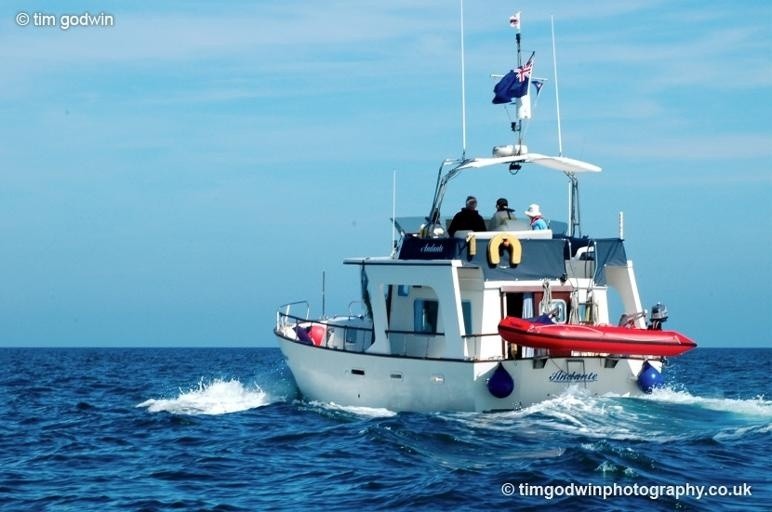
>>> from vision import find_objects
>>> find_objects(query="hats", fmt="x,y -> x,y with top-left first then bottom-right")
466,196 -> 478,209
524,203 -> 542,217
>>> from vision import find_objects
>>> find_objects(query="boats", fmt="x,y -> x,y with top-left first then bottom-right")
494,311 -> 701,359
269,0 -> 666,415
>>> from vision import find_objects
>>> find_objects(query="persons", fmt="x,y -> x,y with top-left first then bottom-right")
418,208 -> 444,237
486,198 -> 517,231
448,195 -> 487,239
523,203 -> 550,232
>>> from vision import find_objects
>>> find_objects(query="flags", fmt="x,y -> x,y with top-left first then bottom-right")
488,54 -> 534,106
